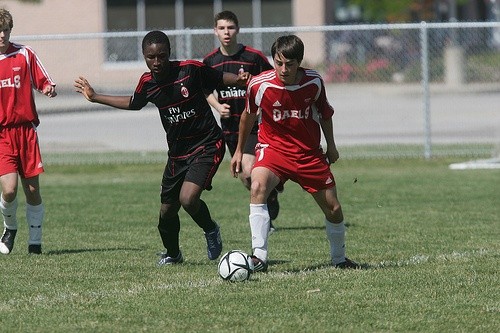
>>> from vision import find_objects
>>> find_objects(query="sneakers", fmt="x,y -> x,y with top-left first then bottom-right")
203,219 -> 222,260
156,250 -> 184,266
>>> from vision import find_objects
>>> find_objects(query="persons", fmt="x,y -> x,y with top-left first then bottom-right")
0,9 -> 56,256
323,0 -> 475,82
199,11 -> 280,236
73,31 -> 252,265
230,34 -> 362,272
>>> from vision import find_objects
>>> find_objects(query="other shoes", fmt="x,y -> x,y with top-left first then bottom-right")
267,189 -> 279,220
330,257 -> 362,270
249,251 -> 269,274
0,228 -> 17,255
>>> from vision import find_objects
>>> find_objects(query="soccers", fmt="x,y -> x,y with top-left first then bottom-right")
218,249 -> 255,282
